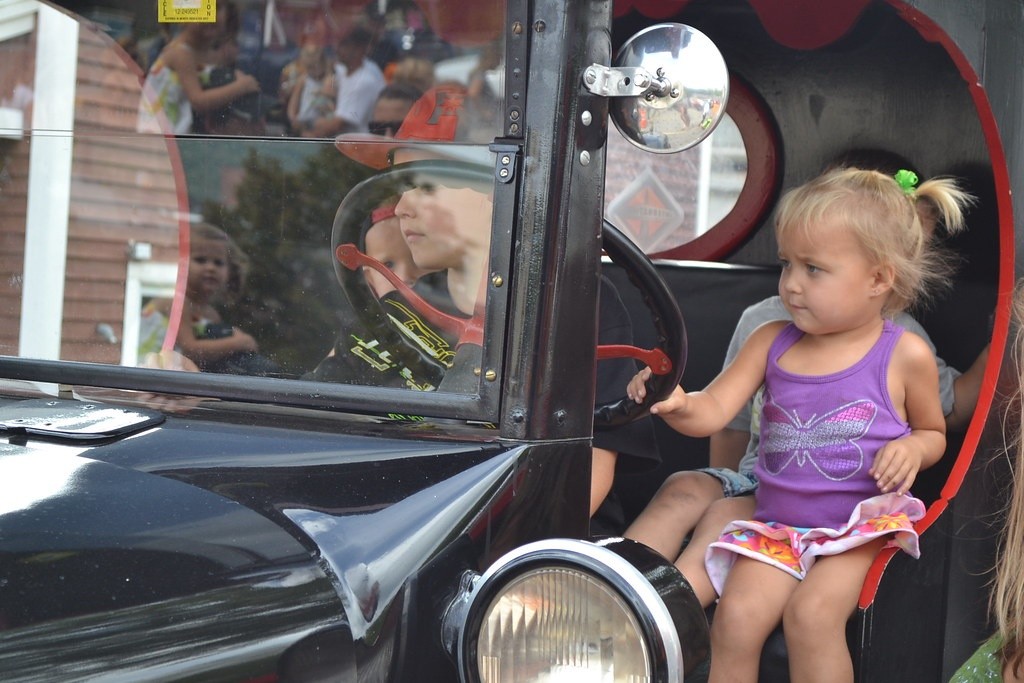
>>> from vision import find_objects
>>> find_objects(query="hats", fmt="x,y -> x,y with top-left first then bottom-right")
369,26 -> 437,72
334,81 -> 496,169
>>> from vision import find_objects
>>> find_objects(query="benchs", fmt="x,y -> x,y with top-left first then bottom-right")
598,257 -> 791,683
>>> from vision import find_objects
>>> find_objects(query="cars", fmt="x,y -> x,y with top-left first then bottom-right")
0,2 -> 1024,682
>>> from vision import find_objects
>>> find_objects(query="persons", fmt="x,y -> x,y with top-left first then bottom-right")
679,98 -> 722,131
295,81 -> 663,521
622,146 -> 992,610
102,0 -> 504,373
626,165 -> 980,683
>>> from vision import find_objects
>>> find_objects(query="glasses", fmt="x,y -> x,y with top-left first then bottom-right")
367,119 -> 407,137
